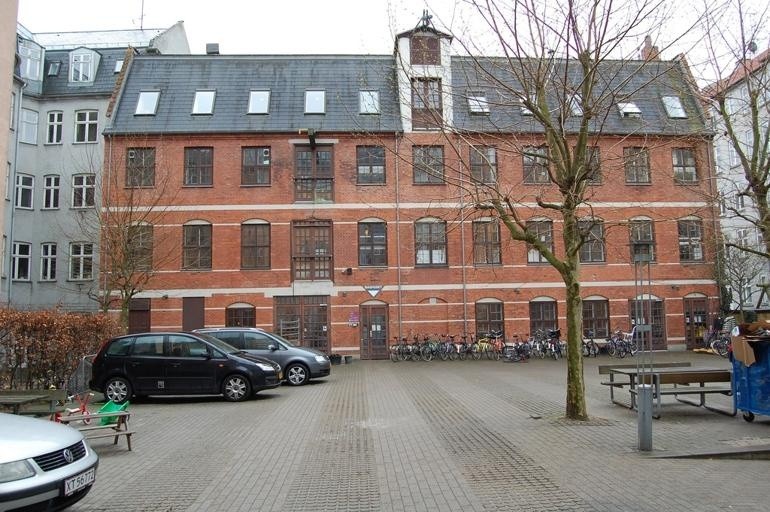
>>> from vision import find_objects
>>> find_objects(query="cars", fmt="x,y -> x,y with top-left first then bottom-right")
0,412 -> 100,512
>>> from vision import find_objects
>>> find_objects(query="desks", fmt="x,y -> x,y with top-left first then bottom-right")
57,411 -> 132,450
0,394 -> 50,414
610,367 -> 728,410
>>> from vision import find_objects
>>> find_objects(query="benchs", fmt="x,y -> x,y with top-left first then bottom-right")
0,389 -> 67,422
598,362 -> 704,409
628,372 -> 737,420
75,424 -> 136,452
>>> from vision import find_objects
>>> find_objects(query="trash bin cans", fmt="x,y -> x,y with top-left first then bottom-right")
345,356 -> 352,364
728,336 -> 770,422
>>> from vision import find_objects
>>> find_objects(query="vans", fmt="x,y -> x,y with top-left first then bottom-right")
88,331 -> 283,404
191,326 -> 332,386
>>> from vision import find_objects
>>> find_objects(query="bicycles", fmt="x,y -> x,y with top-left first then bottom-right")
710,331 -> 731,358
388,330 -> 638,361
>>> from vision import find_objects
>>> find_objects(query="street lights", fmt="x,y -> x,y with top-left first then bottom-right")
626,238 -> 657,452
